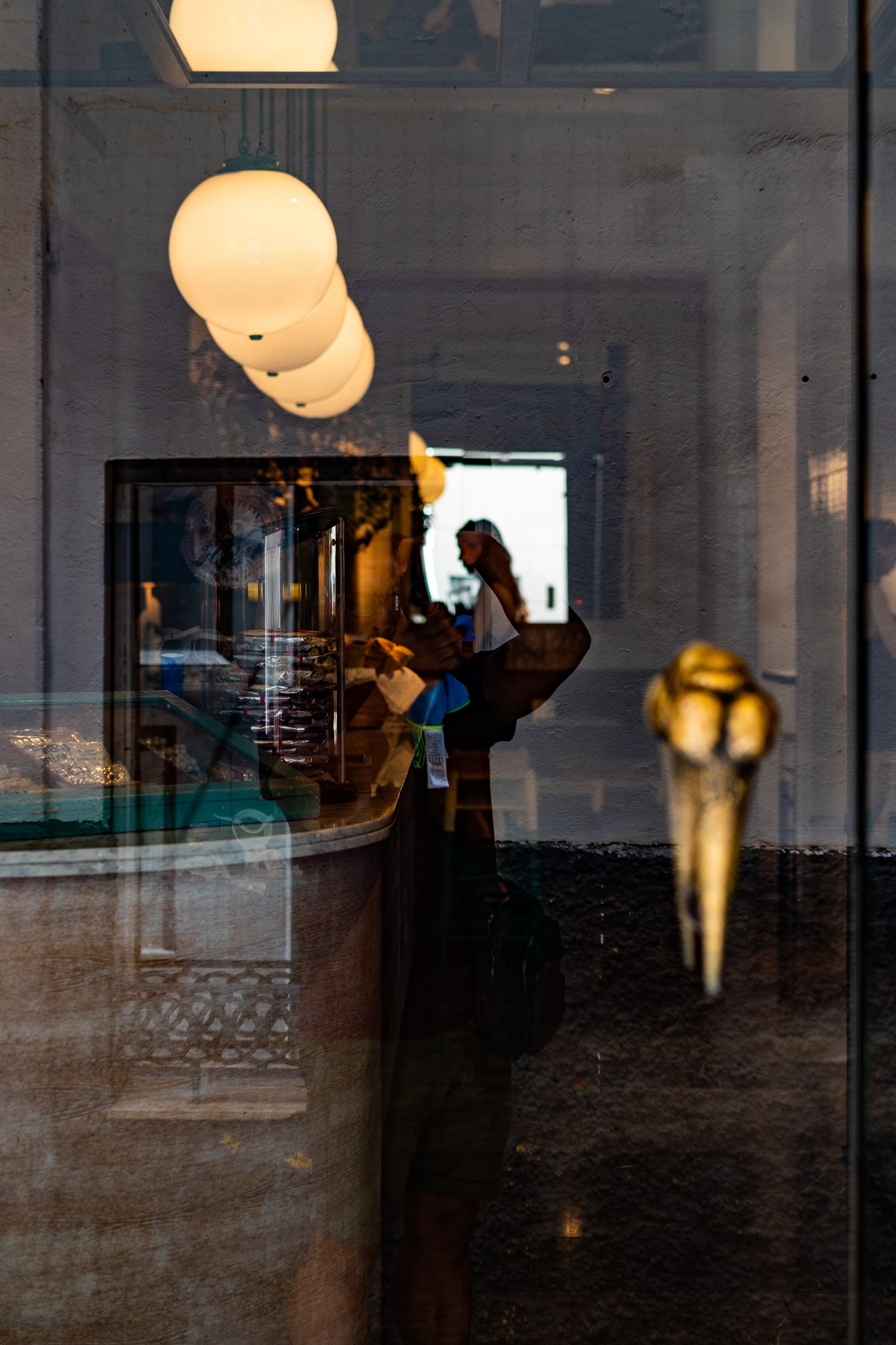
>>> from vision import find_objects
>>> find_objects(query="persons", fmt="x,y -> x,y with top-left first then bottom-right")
433,518 -> 528,745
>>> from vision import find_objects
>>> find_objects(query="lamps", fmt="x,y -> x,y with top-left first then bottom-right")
167,88 -> 374,417
